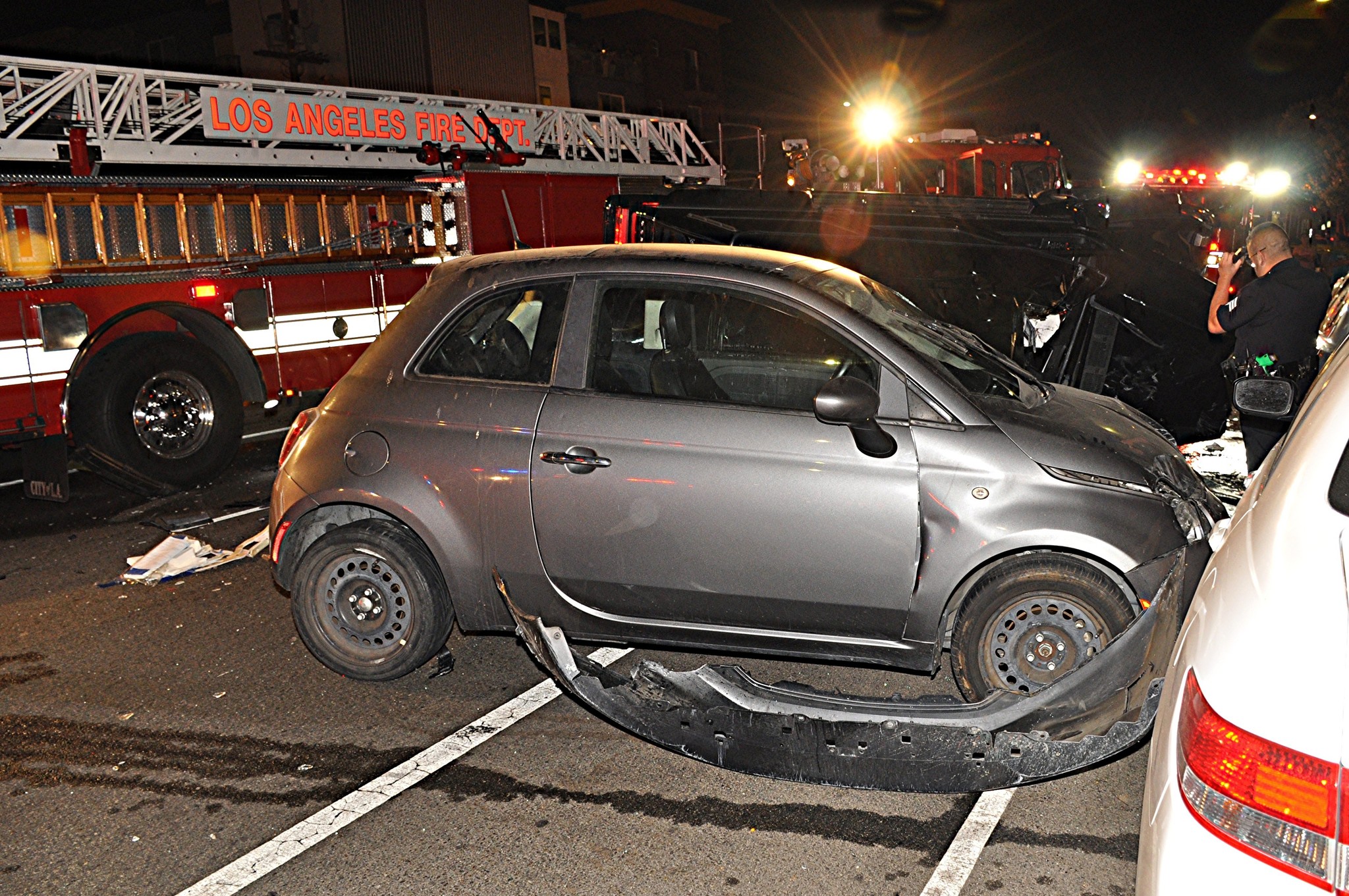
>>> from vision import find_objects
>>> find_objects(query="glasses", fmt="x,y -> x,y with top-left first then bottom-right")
1244,247 -> 1267,267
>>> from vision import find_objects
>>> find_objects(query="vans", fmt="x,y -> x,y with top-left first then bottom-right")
603,189 -> 1232,446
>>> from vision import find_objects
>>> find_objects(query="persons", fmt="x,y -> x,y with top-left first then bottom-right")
1292,233 -> 1322,272
1208,221 -> 1332,477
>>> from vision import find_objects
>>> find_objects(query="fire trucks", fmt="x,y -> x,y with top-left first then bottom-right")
1113,160 -> 1272,231
0,56 -> 1079,510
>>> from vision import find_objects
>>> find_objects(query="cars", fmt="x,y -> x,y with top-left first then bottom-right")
1134,272 -> 1349,896
269,246 -> 1231,707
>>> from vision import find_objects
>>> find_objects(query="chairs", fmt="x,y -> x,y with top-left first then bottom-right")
589,310 -> 637,394
651,301 -> 733,403
